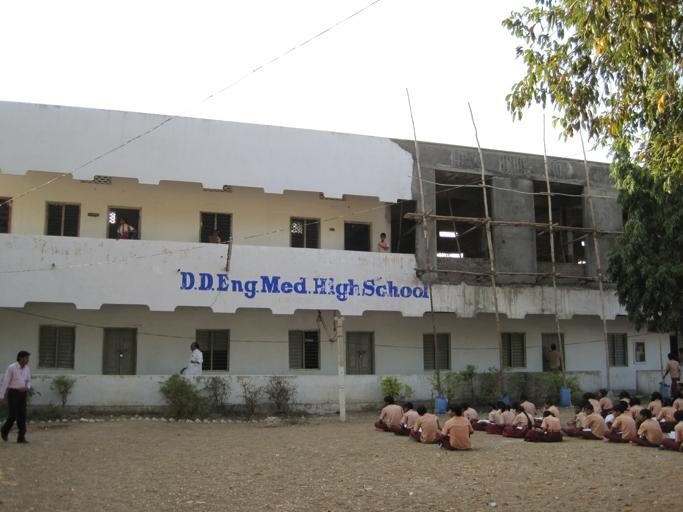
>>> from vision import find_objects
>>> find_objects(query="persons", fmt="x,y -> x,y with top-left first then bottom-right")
440,402 -> 474,450
563,388 -> 683,452
663,353 -> 681,398
375,396 -> 443,444
378,233 -> 389,252
636,347 -> 644,362
180,342 -> 203,375
117,217 -> 135,239
545,345 -> 560,370
461,393 -> 563,441
208,230 -> 225,243
0,351 -> 31,443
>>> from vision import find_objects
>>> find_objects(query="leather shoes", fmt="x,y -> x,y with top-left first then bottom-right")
17,439 -> 29,443
1,428 -> 8,441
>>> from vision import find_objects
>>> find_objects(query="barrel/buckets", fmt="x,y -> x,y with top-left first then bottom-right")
435,396 -> 448,413
560,387 -> 572,405
